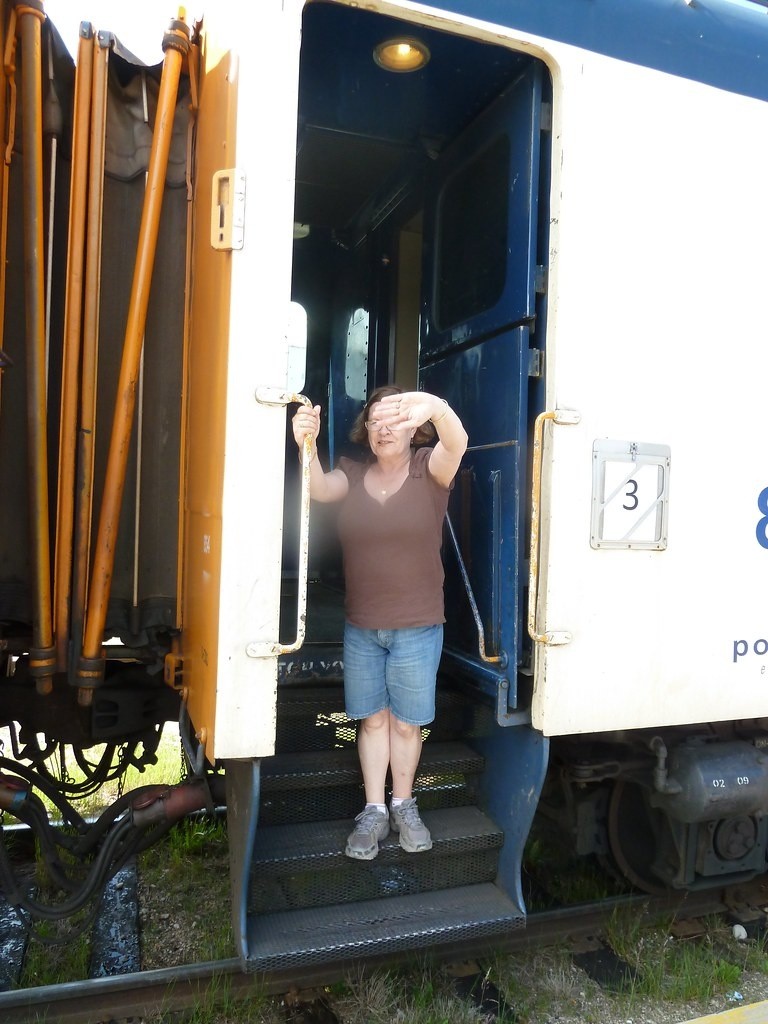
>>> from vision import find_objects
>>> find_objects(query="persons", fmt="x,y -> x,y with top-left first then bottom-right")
292,386 -> 469,859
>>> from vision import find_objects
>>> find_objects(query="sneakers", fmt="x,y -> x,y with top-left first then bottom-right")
389,796 -> 433,853
345,805 -> 391,860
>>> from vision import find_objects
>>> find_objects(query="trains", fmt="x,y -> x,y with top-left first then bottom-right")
0,0 -> 767,1023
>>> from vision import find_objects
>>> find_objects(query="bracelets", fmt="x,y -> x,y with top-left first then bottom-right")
429,399 -> 448,423
298,446 -> 317,463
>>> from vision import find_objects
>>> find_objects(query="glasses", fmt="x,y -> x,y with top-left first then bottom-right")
365,419 -> 401,431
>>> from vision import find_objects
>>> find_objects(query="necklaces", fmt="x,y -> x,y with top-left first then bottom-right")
382,490 -> 386,495
